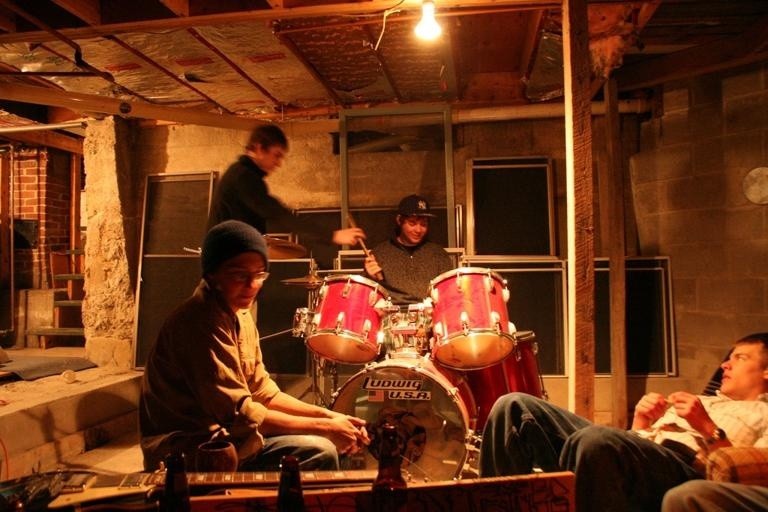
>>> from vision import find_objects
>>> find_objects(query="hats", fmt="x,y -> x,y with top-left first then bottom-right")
201,220 -> 269,278
397,194 -> 438,217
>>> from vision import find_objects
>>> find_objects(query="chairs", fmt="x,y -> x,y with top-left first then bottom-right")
700,332 -> 768,486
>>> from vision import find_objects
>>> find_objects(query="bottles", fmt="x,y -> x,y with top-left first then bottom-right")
159,452 -> 194,511
276,455 -> 308,512
370,424 -> 409,512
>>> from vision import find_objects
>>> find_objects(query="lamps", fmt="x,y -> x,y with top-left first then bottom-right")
414,0 -> 442,41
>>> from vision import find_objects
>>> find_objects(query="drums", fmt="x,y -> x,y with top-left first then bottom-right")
464,330 -> 545,436
293,308 -> 315,338
428,267 -> 518,371
305,275 -> 389,364
330,352 -> 479,483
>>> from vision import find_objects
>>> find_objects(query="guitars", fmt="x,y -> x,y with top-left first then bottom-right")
48,469 -> 412,512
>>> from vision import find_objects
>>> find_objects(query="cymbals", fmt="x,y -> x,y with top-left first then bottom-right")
281,274 -> 323,287
262,233 -> 307,259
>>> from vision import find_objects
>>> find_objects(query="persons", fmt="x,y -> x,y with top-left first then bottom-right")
662,479 -> 768,511
363,195 -> 455,313
138,221 -> 373,470
206,124 -> 369,247
479,332 -> 768,511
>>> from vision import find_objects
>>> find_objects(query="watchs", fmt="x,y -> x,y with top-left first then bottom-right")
706,429 -> 726,444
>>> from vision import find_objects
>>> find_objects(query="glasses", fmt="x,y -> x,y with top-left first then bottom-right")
225,266 -> 269,285
